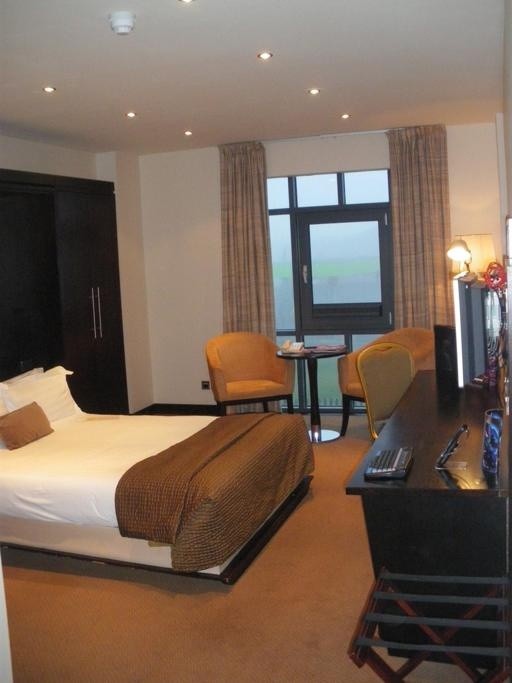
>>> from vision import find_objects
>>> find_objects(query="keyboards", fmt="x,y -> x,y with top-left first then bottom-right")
364,447 -> 414,479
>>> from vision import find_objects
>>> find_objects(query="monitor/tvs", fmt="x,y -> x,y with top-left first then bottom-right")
452,271 -> 487,389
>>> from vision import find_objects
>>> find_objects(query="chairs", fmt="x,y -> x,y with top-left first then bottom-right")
338,327 -> 434,437
356,342 -> 415,440
205,332 -> 295,416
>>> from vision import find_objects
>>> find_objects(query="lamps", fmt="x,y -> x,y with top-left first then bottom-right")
451,234 -> 496,286
446,240 -> 478,286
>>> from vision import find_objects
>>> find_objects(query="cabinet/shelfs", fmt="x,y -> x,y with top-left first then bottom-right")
0,168 -> 131,415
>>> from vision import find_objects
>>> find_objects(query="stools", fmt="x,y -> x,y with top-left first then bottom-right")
347,568 -> 512,683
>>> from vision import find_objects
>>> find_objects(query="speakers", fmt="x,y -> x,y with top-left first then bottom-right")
433,325 -> 457,376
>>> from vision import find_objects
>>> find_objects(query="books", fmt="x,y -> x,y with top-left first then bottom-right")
304,342 -> 347,355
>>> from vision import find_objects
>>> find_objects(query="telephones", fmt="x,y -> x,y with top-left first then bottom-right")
281,340 -> 304,354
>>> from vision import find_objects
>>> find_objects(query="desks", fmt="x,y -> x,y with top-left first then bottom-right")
346,370 -> 512,670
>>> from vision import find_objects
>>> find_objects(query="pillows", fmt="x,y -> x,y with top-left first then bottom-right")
0,365 -> 85,422
0,402 -> 54,451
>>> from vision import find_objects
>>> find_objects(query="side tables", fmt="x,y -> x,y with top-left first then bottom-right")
276,347 -> 347,444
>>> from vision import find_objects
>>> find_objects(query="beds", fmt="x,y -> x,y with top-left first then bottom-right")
0,367 -> 315,585
